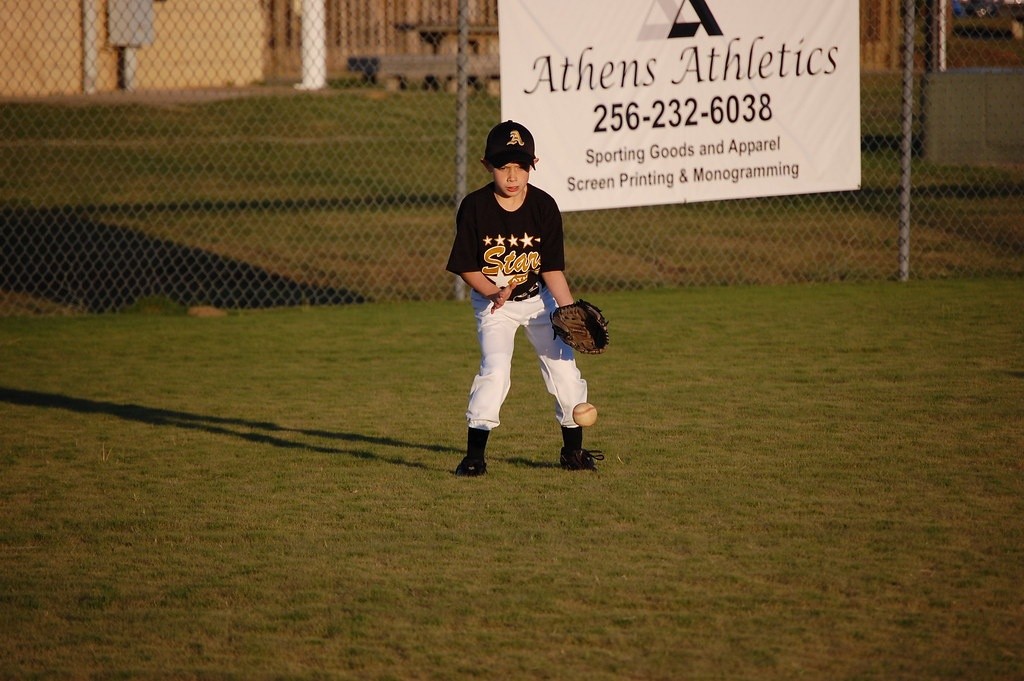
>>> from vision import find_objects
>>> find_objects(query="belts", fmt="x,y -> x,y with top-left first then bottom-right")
508,284 -> 546,302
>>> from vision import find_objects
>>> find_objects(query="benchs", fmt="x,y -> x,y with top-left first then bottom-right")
347,53 -> 500,94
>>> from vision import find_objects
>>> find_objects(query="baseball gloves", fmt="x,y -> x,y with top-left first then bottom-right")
549,298 -> 610,355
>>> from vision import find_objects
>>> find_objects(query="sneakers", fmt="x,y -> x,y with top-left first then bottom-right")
455,458 -> 486,478
559,447 -> 604,471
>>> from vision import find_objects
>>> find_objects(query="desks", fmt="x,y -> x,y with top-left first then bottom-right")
394,23 -> 500,95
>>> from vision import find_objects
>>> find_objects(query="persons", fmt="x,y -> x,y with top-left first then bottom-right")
446,120 -> 604,476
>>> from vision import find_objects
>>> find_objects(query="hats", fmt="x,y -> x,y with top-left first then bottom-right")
484,120 -> 538,170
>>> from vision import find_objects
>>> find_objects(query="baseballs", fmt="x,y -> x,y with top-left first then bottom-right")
572,402 -> 598,428
